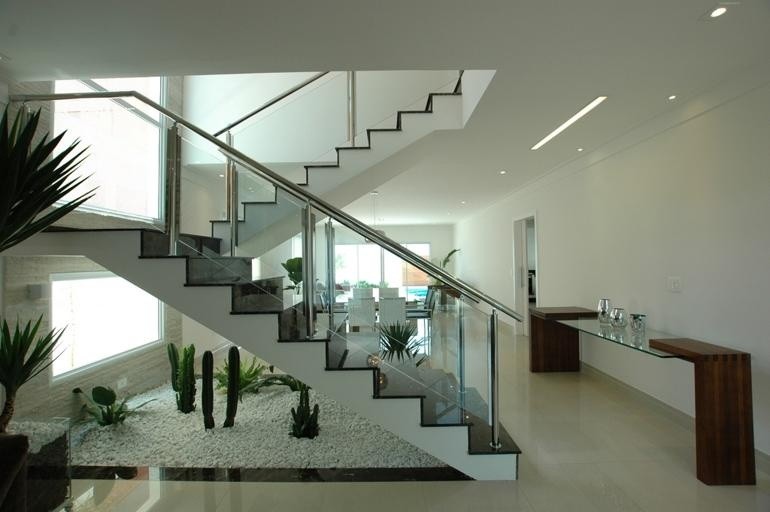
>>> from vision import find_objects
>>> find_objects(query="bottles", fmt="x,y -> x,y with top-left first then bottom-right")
596,298 -> 627,329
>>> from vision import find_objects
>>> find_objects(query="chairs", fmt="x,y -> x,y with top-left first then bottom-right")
348,287 -> 406,332
322,290 -> 348,314
405,288 -> 437,338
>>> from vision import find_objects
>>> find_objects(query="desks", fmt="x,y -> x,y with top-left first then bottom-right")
529,306 -> 757,485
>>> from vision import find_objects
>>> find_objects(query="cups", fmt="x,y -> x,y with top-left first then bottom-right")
629,313 -> 647,337
